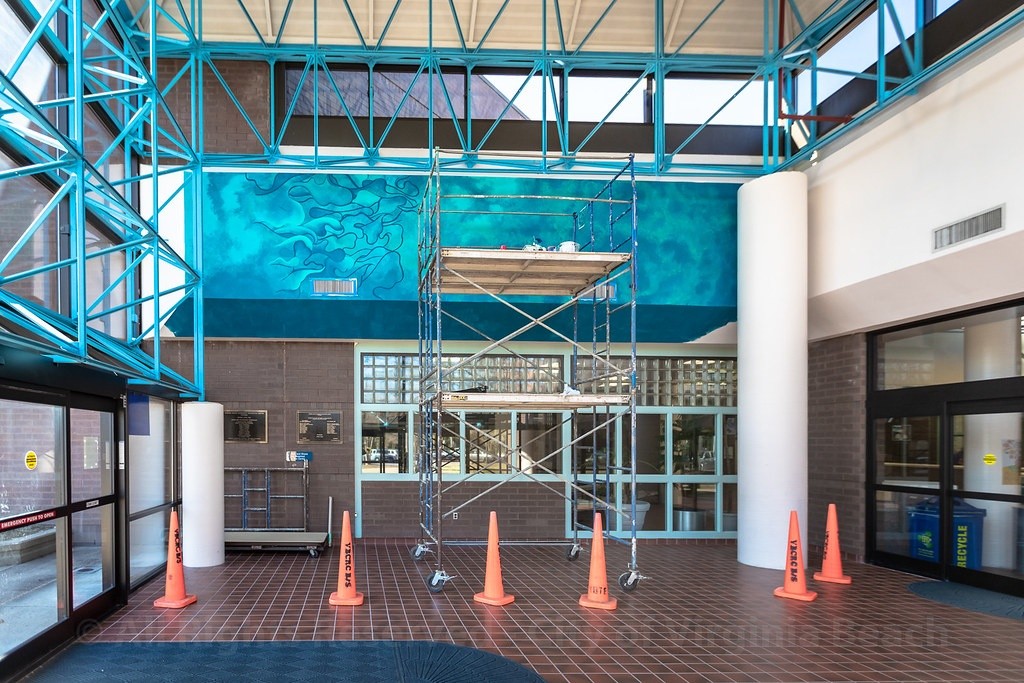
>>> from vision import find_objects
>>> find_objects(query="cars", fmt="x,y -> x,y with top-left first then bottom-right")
585,452 -> 607,467
698,451 -> 714,472
361,449 -> 507,464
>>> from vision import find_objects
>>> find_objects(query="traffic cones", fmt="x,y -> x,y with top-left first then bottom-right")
580,511 -> 617,610
813,504 -> 853,585
774,510 -> 817,601
474,511 -> 514,606
153,510 -> 198,608
328,510 -> 364,607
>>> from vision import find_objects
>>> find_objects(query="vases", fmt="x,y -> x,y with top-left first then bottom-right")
605,500 -> 650,531
723,513 -> 737,531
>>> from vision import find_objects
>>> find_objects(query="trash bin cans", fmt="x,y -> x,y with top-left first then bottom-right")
907,495 -> 986,570
673,507 -> 707,531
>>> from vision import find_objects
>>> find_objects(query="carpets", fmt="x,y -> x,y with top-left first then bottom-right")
908,581 -> 1024,620
25,642 -> 547,683
